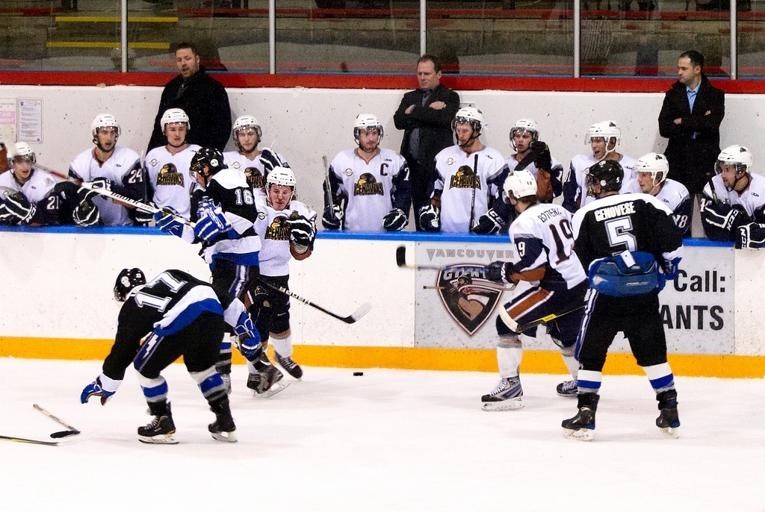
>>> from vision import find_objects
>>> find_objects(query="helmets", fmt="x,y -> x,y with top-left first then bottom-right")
89,113 -> 121,146
633,152 -> 669,187
231,115 -> 262,146
265,166 -> 297,203
502,169 -> 538,205
509,119 -> 541,149
7,141 -> 37,170
160,108 -> 191,135
189,146 -> 228,189
582,159 -> 624,198
353,113 -> 384,140
588,120 -> 621,153
713,145 -> 753,180
451,107 -> 487,134
113,266 -> 146,302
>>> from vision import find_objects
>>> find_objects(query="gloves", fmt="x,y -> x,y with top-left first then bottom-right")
259,147 -> 282,177
383,207 -> 409,231
72,176 -> 114,227
471,208 -> 508,235
322,203 -> 345,230
703,198 -> 765,249
128,198 -> 232,241
418,203 -> 441,232
284,215 -> 315,246
0,191 -> 39,226
484,261 -> 516,283
80,372 -> 123,405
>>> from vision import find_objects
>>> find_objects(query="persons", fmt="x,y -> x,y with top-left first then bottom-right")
418,106 -> 765,249
143,41 -> 232,156
246,165 -> 318,389
658,49 -> 726,238
81,268 -> 237,437
393,53 -> 461,234
0,108 -> 297,229
154,145 -> 284,395
480,168 -> 592,401
561,159 -> 684,429
322,114 -> 411,232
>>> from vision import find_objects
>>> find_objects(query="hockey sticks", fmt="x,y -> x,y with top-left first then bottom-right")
36,404 -> 81,438
397,246 -> 490,275
499,303 -> 587,335
3,137 -> 196,226
259,275 -> 370,322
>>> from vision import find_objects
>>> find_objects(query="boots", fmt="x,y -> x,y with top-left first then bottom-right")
481,376 -> 523,402
137,401 -> 176,437
208,373 -> 236,433
656,389 -> 680,428
247,350 -> 302,394
556,380 -> 600,431
